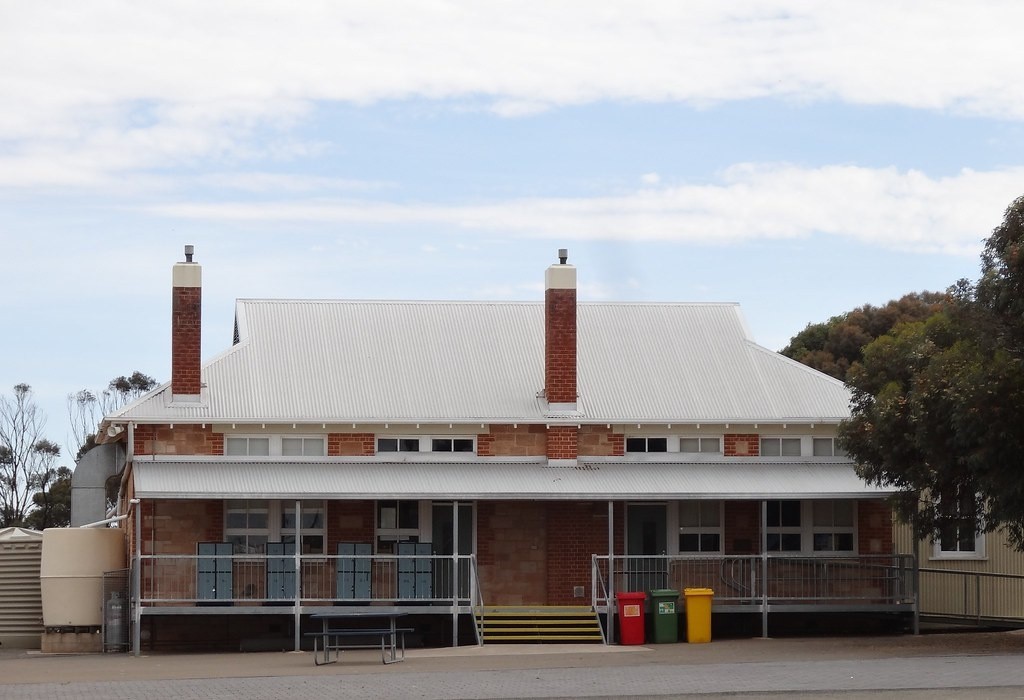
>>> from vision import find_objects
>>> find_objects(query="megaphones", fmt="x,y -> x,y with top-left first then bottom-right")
107,427 -> 124,437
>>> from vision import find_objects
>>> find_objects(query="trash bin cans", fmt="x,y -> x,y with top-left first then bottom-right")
683,588 -> 715,644
648,589 -> 680,644
615,592 -> 647,645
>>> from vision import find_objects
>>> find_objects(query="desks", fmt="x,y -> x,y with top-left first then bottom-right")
309,612 -> 408,667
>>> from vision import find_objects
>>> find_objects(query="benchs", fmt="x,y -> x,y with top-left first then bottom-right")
303,631 -> 395,664
318,628 -> 415,659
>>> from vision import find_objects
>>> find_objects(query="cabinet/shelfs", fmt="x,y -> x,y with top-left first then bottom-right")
334,542 -> 372,607
262,542 -> 301,605
196,541 -> 233,607
393,542 -> 435,606
